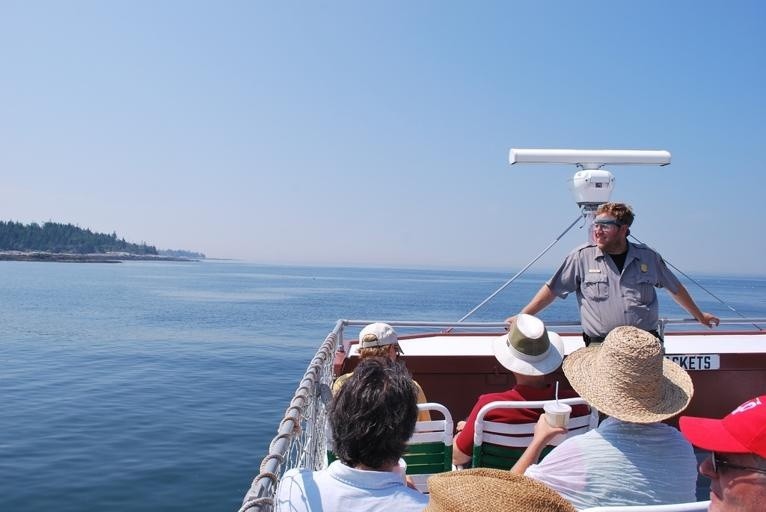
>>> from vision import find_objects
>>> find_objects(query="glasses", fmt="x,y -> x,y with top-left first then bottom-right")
592,224 -> 611,231
711,451 -> 766,475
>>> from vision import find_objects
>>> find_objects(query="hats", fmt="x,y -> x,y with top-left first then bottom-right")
679,395 -> 766,460
358,323 -> 403,354
562,325 -> 695,423
491,314 -> 564,376
424,468 -> 578,512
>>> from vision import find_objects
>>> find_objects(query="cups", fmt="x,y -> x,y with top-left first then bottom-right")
393,456 -> 409,487
542,404 -> 574,446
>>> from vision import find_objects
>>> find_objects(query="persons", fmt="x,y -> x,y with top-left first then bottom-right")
506,326 -> 700,509
505,202 -> 722,349
330,321 -> 432,420
677,393 -> 766,512
268,356 -> 429,512
451,311 -> 591,470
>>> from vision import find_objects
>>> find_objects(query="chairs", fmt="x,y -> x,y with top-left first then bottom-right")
324,397 -> 600,500
